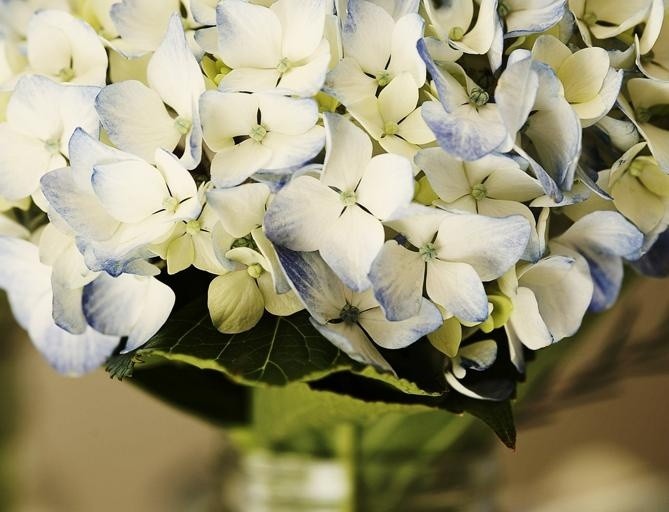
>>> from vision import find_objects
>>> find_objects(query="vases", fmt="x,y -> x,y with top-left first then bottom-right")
217,432 -> 478,510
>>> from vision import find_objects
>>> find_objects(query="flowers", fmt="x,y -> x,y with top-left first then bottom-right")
2,2 -> 667,511
